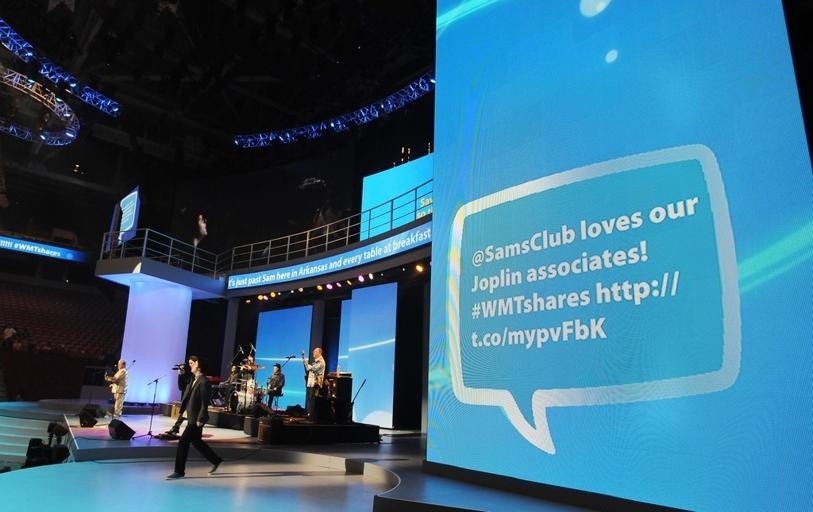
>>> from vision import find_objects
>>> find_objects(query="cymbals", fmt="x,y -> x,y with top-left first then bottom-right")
238,364 -> 248,369
230,382 -> 241,386
251,365 -> 265,370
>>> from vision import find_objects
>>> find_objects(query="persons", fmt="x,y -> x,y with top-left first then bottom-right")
165,358 -> 224,478
178,207 -> 208,267
1,323 -> 15,346
302,347 -> 326,415
220,365 -> 239,402
267,362 -> 284,409
103,359 -> 127,418
164,355 -> 194,433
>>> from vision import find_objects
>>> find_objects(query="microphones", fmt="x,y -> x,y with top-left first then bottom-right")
250,344 -> 256,352
285,356 -> 296,359
239,345 -> 244,355
172,365 -> 185,370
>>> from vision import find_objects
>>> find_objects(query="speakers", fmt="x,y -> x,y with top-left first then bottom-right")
108,418 -> 136,440
82,403 -> 101,418
79,410 -> 98,428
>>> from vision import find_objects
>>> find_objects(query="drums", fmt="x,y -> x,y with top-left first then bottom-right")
237,378 -> 246,391
256,387 -> 268,395
246,379 -> 257,392
229,392 -> 254,413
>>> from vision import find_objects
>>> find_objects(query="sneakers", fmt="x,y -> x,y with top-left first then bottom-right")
207,458 -> 224,475
166,472 -> 184,480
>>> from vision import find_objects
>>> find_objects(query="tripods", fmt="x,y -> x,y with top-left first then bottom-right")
132,370 -> 174,439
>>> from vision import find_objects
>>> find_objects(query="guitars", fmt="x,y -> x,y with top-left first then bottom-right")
301,350 -> 309,387
